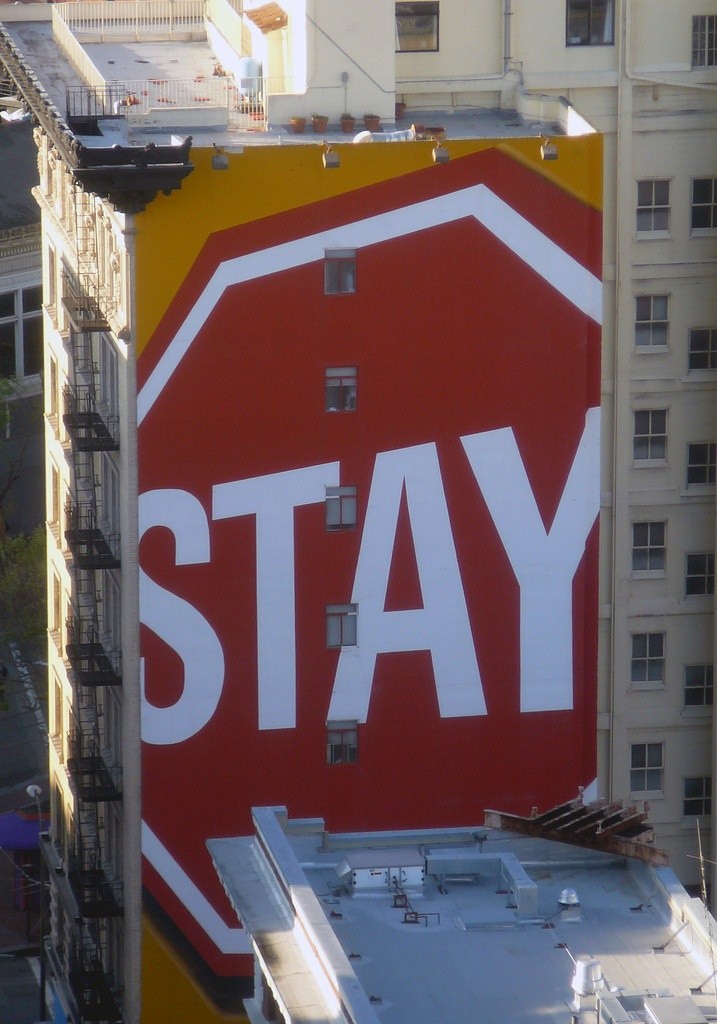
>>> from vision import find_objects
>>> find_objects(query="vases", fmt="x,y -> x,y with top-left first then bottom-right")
395,103 -> 407,119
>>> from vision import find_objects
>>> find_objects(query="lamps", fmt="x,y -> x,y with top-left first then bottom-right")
321,140 -> 339,168
430,138 -> 449,162
211,142 -> 229,170
538,136 -> 559,161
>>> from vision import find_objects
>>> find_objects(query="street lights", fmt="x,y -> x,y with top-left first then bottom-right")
27,785 -> 48,1024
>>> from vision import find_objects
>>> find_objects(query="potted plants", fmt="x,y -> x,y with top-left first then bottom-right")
363,113 -> 381,132
312,113 -> 328,133
341,113 -> 355,133
291,116 -> 307,133
